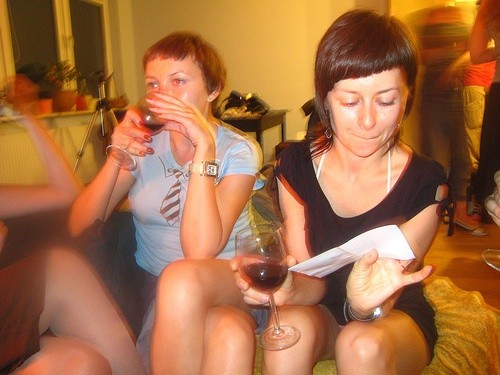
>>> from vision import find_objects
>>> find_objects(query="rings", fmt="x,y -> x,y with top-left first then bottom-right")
399,260 -> 405,268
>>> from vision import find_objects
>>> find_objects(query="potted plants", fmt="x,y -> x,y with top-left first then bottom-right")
76,72 -> 93,111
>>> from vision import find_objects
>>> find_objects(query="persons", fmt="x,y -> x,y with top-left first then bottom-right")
67,31 -> 274,374
403,0 -> 500,236
0,73 -> 148,375
229,9 -> 451,374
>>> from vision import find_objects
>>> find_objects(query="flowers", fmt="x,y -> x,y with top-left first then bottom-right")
44,59 -> 79,90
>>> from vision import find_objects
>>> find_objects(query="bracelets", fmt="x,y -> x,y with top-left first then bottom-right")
347,305 -> 381,321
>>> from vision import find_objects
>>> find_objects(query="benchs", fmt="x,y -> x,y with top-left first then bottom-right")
252,274 -> 500,375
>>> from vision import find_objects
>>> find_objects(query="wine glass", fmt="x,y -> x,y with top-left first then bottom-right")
105,92 -> 169,171
234,223 -> 301,351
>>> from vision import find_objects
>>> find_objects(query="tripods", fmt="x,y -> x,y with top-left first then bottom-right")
71,69 -> 119,172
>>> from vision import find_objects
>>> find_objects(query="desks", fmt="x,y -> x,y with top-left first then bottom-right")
222,110 -> 288,163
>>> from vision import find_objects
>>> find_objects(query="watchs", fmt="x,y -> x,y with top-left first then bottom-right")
188,161 -> 219,178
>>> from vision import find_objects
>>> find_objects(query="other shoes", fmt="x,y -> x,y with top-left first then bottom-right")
454,220 -> 488,235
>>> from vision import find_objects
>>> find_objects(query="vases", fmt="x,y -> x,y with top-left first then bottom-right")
52,90 -> 77,112
